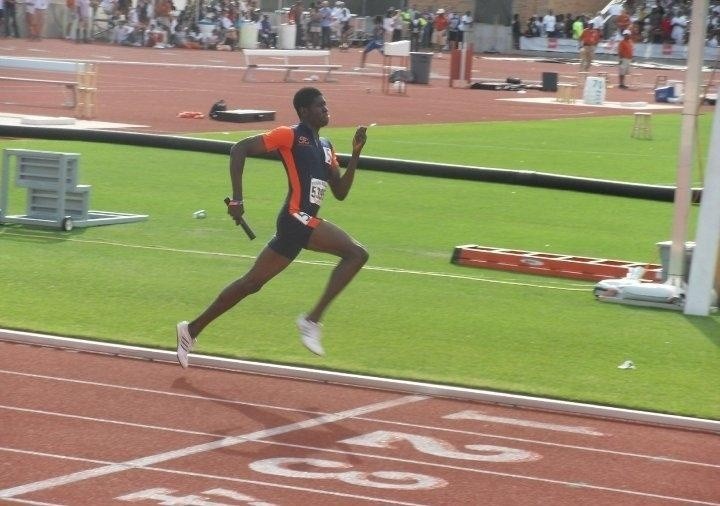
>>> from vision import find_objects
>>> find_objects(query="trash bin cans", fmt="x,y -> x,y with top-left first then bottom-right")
659,242 -> 695,283
411,53 -> 433,83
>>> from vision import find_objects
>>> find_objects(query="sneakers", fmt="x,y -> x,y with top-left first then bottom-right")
296,312 -> 325,356
176,321 -> 197,371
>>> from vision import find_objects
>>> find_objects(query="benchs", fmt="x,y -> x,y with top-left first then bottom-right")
1,55 -> 93,108
241,47 -> 342,82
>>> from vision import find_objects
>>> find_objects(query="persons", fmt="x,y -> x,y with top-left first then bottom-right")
1,0 -> 279,51
175,88 -> 369,369
288,0 -> 474,69
511,1 -> 720,88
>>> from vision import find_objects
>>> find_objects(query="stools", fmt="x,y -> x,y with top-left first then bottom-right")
555,72 -> 669,102
631,112 -> 654,140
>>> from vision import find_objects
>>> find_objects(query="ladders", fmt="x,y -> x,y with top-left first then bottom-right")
451,244 -> 666,285
